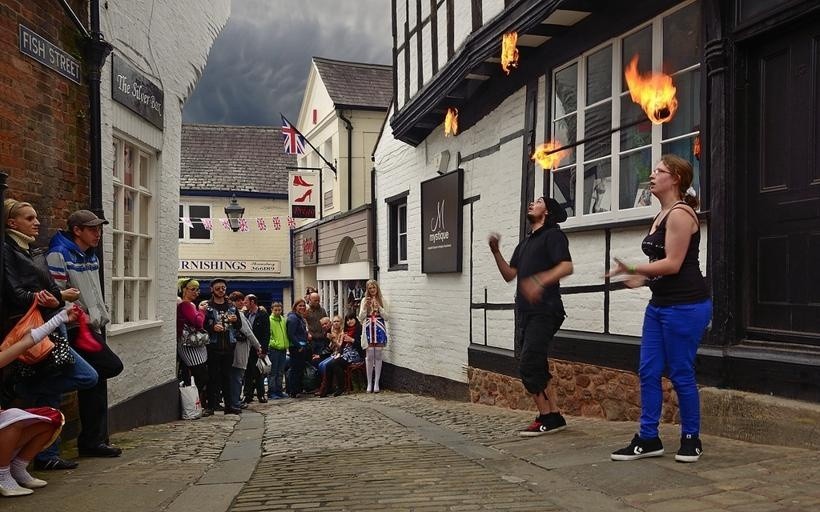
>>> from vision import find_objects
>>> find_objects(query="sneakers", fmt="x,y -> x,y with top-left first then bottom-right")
32,456 -> 78,470
518,411 -> 568,437
610,433 -> 665,461
675,432 -> 703,463
269,392 -> 305,400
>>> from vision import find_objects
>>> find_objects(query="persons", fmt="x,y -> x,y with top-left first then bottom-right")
175,276 -> 365,417
47,209 -> 123,458
0,303 -> 80,497
487,197 -> 574,439
356,279 -> 388,394
602,152 -> 714,462
0,199 -> 98,468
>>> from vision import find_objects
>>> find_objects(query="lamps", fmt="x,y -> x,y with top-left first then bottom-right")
224,193 -> 245,233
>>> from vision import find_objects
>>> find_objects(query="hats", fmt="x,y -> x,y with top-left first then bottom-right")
211,278 -> 227,286
67,209 -> 109,228
540,196 -> 568,224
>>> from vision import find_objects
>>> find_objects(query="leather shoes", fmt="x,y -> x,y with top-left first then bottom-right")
257,394 -> 268,403
1,472 -> 47,497
78,442 -> 122,458
366,388 -> 380,393
203,396 -> 252,416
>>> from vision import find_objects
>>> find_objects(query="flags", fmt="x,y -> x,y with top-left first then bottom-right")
180,218 -> 195,229
287,216 -> 295,229
237,218 -> 248,233
280,115 -> 305,155
200,218 -> 213,230
219,218 -> 232,231
272,217 -> 281,231
256,218 -> 267,232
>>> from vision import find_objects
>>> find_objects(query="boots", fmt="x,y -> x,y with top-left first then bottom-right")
315,379 -> 343,396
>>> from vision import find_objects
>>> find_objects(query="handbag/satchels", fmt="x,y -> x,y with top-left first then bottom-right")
343,347 -> 361,363
235,329 -> 248,341
256,354 -> 273,377
18,333 -> 75,377
303,367 -> 318,379
182,324 -> 211,347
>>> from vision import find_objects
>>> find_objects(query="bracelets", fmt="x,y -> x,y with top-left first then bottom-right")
626,262 -> 636,275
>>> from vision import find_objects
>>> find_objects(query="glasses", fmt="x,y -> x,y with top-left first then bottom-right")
215,286 -> 226,291
189,288 -> 199,292
654,167 -> 671,176
